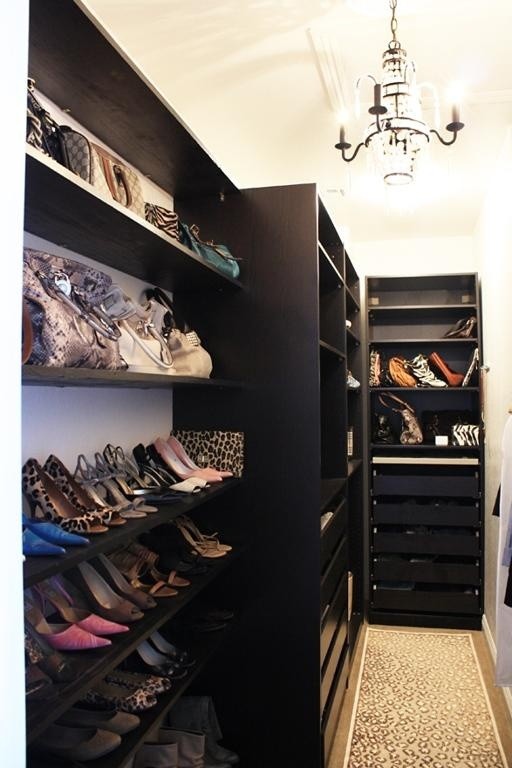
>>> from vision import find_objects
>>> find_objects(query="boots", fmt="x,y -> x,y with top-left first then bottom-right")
135,695 -> 240,767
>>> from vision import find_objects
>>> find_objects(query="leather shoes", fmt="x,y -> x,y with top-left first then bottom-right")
39,598 -> 235,763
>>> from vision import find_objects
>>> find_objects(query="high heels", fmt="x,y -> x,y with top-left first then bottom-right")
20,434 -> 234,561
26,514 -> 236,701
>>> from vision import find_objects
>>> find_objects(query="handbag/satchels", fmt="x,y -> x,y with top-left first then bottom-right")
369,346 -> 477,388
59,126 -> 145,220
26,78 -> 61,164
444,315 -> 477,338
23,246 -> 215,380
374,393 -> 480,447
170,427 -> 246,477
143,202 -> 185,240
178,217 -> 244,281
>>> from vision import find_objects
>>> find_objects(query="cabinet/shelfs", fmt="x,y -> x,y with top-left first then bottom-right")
170,182 -> 365,768
22,0 -> 241,766
361,269 -> 486,633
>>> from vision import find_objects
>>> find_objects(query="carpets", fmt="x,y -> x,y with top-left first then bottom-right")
341,625 -> 510,768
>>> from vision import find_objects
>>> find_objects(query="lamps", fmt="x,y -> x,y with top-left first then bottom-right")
330,0 -> 466,187
